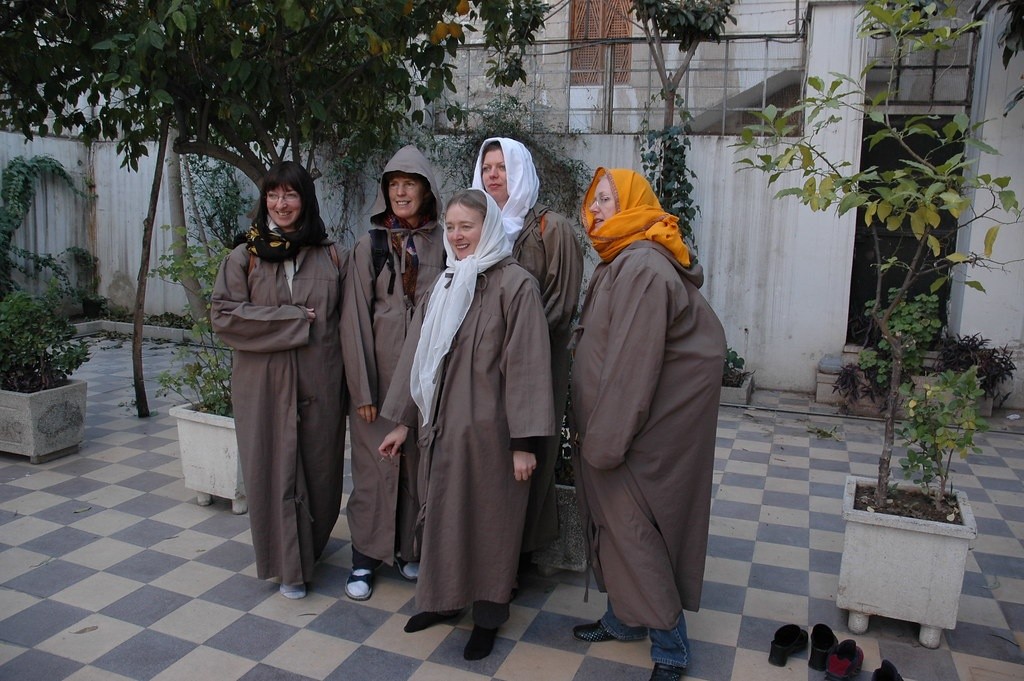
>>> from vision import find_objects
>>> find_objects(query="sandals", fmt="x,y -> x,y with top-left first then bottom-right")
345,563 -> 376,600
394,550 -> 418,580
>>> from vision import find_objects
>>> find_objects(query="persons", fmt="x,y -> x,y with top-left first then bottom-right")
571,166 -> 727,681
212,161 -> 350,599
378,188 -> 556,660
339,145 -> 447,600
471,137 -> 584,603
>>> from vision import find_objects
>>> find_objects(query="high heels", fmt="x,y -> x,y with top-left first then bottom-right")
808,623 -> 838,672
768,624 -> 809,667
871,659 -> 903,681
826,639 -> 864,681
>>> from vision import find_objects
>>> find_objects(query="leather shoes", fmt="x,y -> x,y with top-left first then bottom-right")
649,662 -> 684,681
572,621 -> 618,641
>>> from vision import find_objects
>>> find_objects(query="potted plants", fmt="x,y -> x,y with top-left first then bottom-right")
51,248 -> 110,319
0,272 -> 95,463
524,316 -> 588,575
724,0 -> 1024,648
147,221 -> 249,515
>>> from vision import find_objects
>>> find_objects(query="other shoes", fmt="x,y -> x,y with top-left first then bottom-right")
279,583 -> 306,600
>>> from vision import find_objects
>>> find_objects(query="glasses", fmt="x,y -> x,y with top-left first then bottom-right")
593,196 -> 616,206
266,192 -> 300,201
